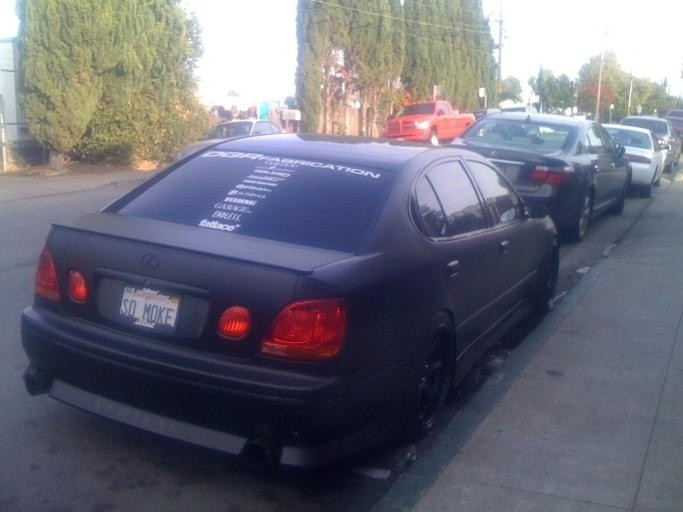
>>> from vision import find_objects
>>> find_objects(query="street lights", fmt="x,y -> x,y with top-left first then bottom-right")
478,87 -> 488,108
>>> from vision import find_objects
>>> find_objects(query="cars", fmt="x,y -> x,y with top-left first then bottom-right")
17,127 -> 563,483
